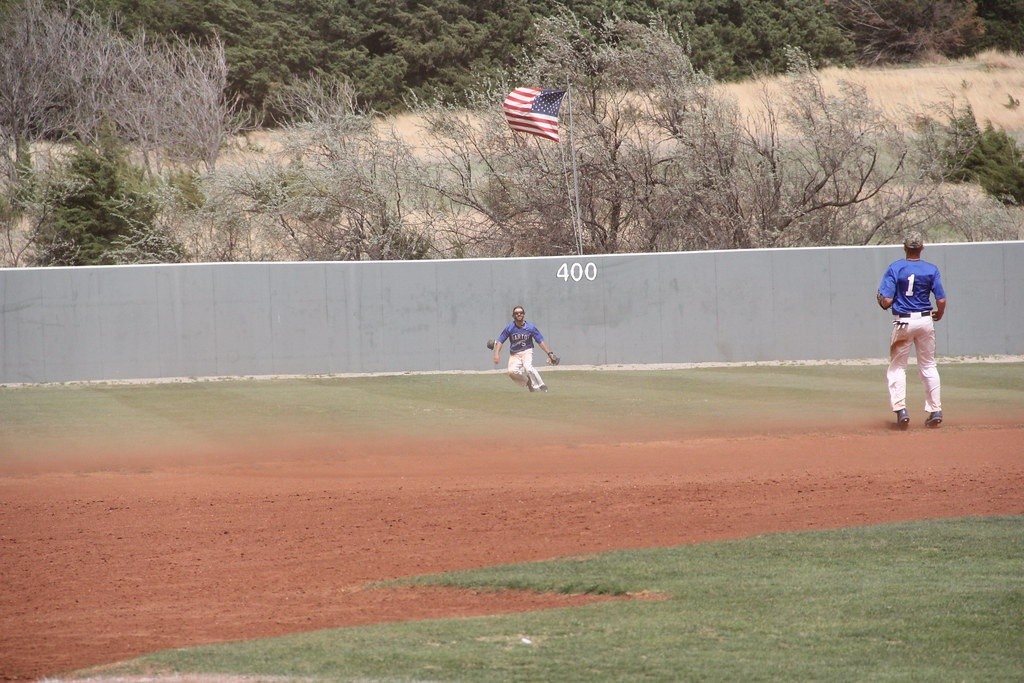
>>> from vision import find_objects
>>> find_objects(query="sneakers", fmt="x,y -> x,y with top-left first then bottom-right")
925,411 -> 942,427
897,409 -> 909,429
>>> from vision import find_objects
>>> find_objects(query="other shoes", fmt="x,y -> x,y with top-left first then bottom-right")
526,375 -> 534,392
540,385 -> 547,392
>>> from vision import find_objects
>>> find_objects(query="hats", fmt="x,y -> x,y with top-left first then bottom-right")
903,231 -> 923,248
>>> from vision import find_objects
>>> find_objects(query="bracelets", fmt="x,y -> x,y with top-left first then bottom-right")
548,352 -> 553,356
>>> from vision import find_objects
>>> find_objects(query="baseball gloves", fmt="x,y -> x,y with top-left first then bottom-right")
546,352 -> 560,366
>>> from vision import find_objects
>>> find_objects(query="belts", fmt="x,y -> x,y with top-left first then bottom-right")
899,311 -> 930,317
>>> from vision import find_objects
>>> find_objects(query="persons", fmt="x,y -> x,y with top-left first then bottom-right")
877,231 -> 947,428
494,306 -> 561,393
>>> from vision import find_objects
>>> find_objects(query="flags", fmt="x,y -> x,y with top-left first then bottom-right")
503,86 -> 567,143
